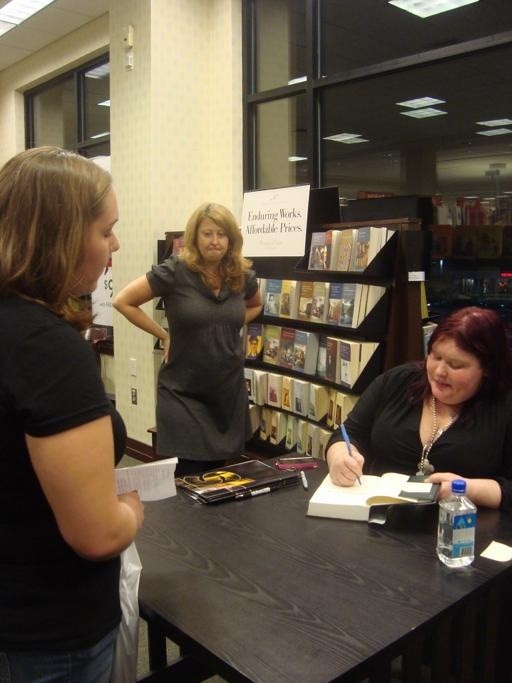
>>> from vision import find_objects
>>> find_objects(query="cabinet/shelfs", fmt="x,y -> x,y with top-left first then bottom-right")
155,214 -> 422,463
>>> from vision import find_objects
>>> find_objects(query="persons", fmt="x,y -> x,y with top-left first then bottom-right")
112,203 -> 262,477
0,144 -> 144,683
325,306 -> 512,683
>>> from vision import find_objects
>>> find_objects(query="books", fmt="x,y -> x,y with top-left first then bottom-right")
173,238 -> 178,255
242,226 -> 396,460
305,472 -> 440,525
175,460 -> 300,505
178,236 -> 184,249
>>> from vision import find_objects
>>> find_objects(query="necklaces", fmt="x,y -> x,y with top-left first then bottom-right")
415,398 -> 460,475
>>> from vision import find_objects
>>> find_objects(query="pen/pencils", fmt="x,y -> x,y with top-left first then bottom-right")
300,470 -> 308,488
279,456 -> 313,461
235,485 -> 282,499
340,423 -> 362,485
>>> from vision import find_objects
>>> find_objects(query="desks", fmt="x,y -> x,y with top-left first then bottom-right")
126,444 -> 512,682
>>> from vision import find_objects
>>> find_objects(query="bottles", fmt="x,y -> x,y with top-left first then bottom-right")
436,479 -> 478,569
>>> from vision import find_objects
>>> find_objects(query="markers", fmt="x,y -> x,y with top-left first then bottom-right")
277,463 -> 319,470
